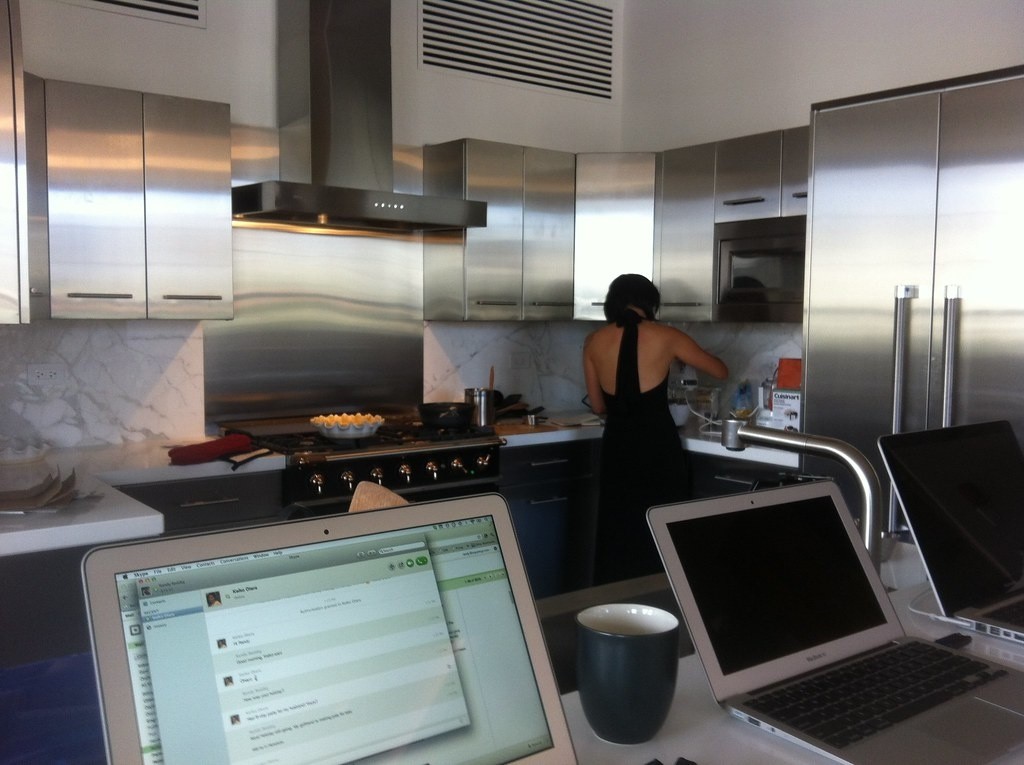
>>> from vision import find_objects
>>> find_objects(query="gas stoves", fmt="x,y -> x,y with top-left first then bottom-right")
217,407 -> 509,509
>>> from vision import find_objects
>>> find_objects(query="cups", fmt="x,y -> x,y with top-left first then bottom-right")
523,414 -> 536,426
465,387 -> 496,426
723,419 -> 747,451
571,603 -> 679,745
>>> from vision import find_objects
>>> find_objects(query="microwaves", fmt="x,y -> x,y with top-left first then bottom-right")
712,215 -> 802,323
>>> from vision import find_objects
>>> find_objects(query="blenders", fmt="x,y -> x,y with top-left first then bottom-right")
669,358 -> 699,428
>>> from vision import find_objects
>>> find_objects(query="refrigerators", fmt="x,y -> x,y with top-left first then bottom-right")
801,65 -> 1024,545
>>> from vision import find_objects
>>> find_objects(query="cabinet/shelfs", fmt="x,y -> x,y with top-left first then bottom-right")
496,439 -> 595,599
573,151 -> 656,323
423,137 -> 574,323
111,469 -> 289,536
693,452 -> 802,498
23,70 -> 50,322
42,77 -> 234,322
714,125 -> 810,243
657,142 -> 735,324
0,0 -> 31,326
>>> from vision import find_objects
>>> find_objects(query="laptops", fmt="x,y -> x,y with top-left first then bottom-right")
647,479 -> 1024,765
80,492 -> 580,765
878,420 -> 1024,643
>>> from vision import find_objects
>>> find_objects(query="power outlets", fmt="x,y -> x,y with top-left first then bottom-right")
27,363 -> 66,387
508,351 -> 531,369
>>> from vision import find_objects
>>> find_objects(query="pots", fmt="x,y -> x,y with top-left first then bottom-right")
418,402 -> 476,427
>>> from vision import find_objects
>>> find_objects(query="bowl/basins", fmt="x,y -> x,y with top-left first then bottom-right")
309,412 -> 385,439
669,405 -> 692,426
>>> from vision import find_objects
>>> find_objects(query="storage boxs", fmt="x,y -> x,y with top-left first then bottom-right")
771,387 -> 801,432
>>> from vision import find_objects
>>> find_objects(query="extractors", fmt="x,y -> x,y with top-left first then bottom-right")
232,0 -> 488,240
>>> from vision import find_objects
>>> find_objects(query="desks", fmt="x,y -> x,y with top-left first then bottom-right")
561,581 -> 1024,765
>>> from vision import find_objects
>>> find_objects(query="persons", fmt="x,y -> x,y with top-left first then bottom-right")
582,273 -> 727,584
